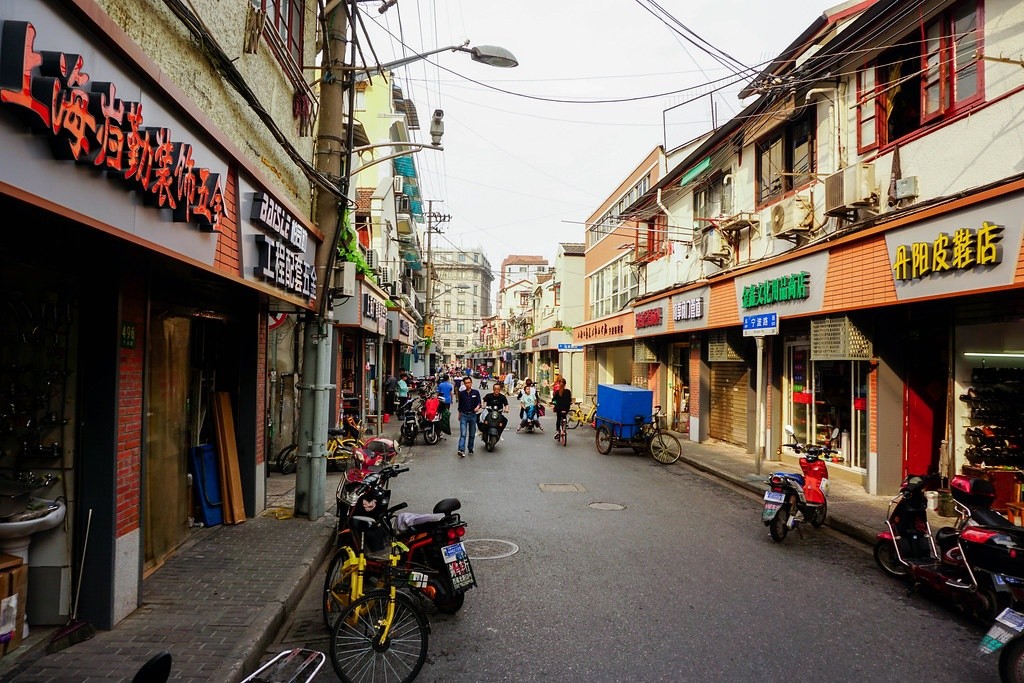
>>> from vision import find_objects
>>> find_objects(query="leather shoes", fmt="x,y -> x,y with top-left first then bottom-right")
959,367 -> 1023,469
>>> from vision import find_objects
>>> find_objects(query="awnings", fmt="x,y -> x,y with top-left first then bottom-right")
393,85 -> 420,130
404,251 -> 421,271
393,156 -> 425,224
342,122 -> 373,156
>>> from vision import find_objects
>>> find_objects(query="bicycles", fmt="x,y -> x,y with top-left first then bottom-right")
549,402 -> 569,448
565,394 -> 599,428
277,426 -> 367,476
321,501 -> 434,683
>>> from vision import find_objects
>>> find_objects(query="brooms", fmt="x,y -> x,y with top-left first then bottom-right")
46,508 -> 64,655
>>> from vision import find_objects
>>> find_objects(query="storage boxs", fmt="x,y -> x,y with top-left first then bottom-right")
410,572 -> 428,588
951,475 -> 996,507
595,384 -> 654,438
0,552 -> 28,657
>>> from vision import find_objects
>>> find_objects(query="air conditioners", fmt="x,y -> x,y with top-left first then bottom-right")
394,174 -> 410,211
771,195 -> 810,236
368,249 -> 380,276
697,226 -> 729,261
825,163 -> 876,215
380,266 -> 393,286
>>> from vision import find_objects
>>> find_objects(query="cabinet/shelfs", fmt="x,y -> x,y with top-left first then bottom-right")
961,380 -> 1024,512
794,402 -> 841,449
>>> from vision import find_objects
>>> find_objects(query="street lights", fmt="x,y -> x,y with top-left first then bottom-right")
423,284 -> 470,377
296,43 -> 519,518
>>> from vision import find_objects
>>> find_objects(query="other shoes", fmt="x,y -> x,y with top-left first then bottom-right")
540,426 -> 545,432
399,416 -> 405,421
501,437 -> 504,441
554,432 -> 559,439
469,450 -> 473,453
517,426 -> 521,432
458,451 -> 466,457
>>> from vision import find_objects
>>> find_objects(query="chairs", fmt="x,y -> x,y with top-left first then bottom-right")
366,398 -> 383,434
1006,483 -> 1024,528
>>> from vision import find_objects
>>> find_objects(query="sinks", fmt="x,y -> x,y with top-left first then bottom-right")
0,496 -> 66,539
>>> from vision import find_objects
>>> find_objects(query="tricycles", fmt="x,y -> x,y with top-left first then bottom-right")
594,405 -> 684,465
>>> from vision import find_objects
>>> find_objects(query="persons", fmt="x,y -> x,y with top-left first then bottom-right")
385,367 -> 412,420
517,386 -> 545,432
436,375 -> 453,410
517,379 -> 539,419
551,375 -> 562,405
448,364 -> 490,402
550,378 -> 571,439
481,383 -> 509,442
458,377 -> 481,457
504,372 -> 515,396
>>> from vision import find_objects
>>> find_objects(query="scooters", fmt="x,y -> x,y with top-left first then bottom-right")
480,406 -> 509,453
478,376 -> 490,391
336,436 -> 400,527
760,425 -> 843,543
335,437 -> 481,616
395,374 -> 447,446
870,470 -> 1024,683
526,405 -> 541,434
